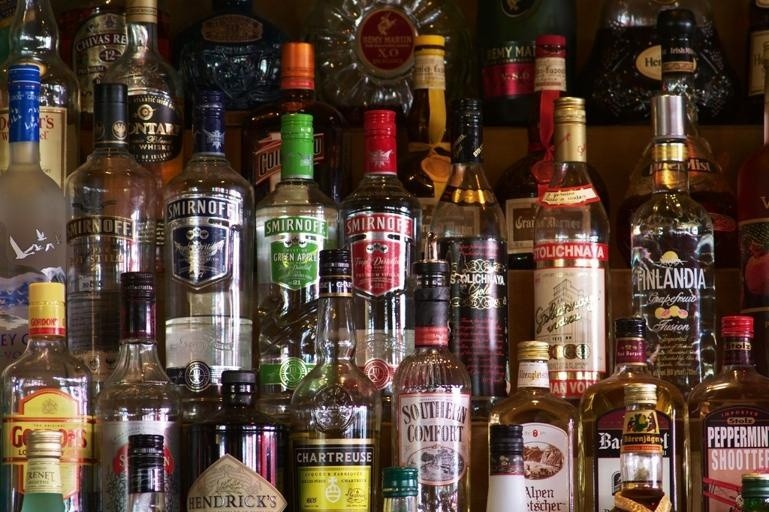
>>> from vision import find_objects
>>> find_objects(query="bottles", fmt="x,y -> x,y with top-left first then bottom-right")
1,1 -> 768,512
